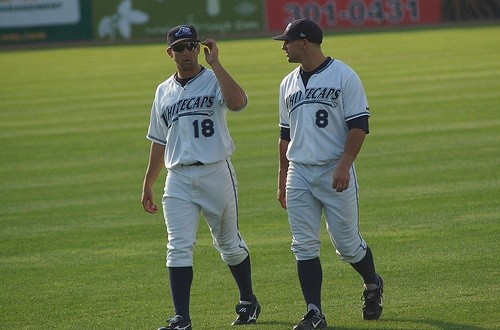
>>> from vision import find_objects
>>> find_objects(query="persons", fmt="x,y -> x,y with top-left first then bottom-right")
271,17 -> 384,330
141,25 -> 260,330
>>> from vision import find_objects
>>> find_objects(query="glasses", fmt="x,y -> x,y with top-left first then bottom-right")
170,43 -> 198,52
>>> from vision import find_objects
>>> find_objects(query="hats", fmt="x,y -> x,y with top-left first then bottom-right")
167,24 -> 200,49
272,17 -> 322,43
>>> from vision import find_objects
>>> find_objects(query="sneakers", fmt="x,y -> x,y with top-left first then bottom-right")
231,295 -> 261,326
156,315 -> 192,330
292,303 -> 327,330
361,274 -> 384,320
